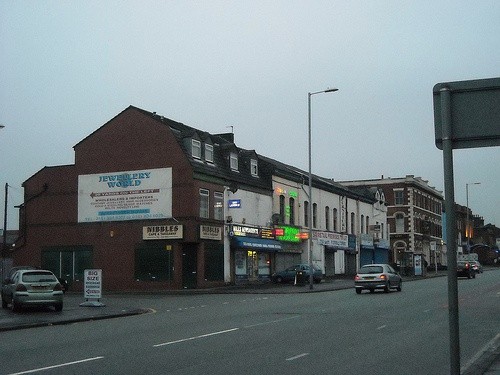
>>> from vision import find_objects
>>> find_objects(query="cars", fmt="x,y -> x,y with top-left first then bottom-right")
3,269 -> 64,313
457,261 -> 476,279
354,264 -> 402,294
272,264 -> 322,284
469,259 -> 484,274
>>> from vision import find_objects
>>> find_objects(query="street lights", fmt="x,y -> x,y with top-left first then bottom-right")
307,87 -> 339,287
466,182 -> 485,253
